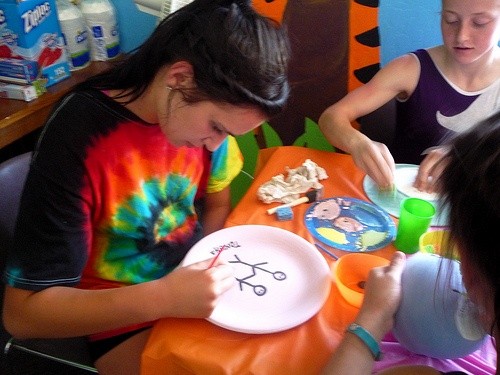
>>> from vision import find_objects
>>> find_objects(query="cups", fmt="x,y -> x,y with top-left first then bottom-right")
393,197 -> 437,253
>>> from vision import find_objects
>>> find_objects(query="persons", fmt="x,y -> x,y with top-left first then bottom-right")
317,110 -> 500,375
0,0 -> 292,358
316,0 -> 500,192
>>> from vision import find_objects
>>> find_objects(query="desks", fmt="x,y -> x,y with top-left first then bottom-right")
96,146 -> 450,375
0,53 -> 134,149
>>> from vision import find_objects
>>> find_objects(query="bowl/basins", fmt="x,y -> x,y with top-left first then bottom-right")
418,229 -> 459,260
334,252 -> 391,309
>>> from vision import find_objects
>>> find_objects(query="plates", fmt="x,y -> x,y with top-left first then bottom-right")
303,196 -> 397,253
363,164 -> 451,227
181,224 -> 333,335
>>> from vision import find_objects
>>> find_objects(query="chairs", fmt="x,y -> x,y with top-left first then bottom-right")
0,152 -> 101,375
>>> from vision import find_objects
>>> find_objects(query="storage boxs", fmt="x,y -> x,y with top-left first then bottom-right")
0,0 -> 71,102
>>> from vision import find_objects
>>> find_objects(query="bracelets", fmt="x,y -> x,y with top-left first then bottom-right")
345,323 -> 382,361
